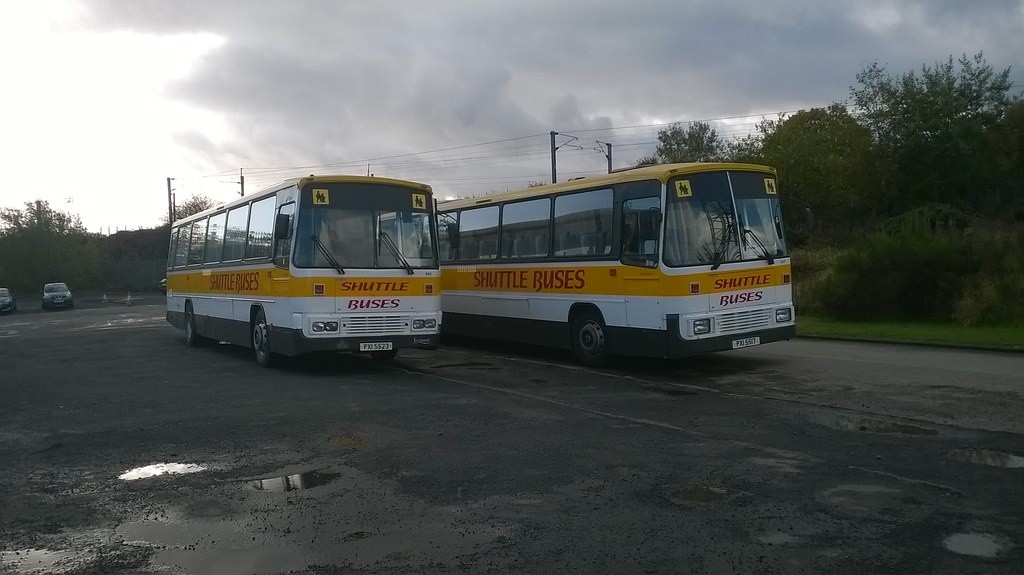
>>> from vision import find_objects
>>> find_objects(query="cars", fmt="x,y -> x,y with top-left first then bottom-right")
0,288 -> 17,315
40,282 -> 73,310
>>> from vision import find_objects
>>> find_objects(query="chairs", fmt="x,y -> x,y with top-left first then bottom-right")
447,229 -> 639,259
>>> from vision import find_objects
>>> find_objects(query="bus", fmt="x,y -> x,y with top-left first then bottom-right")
376,162 -> 798,366
166,175 -> 445,368
